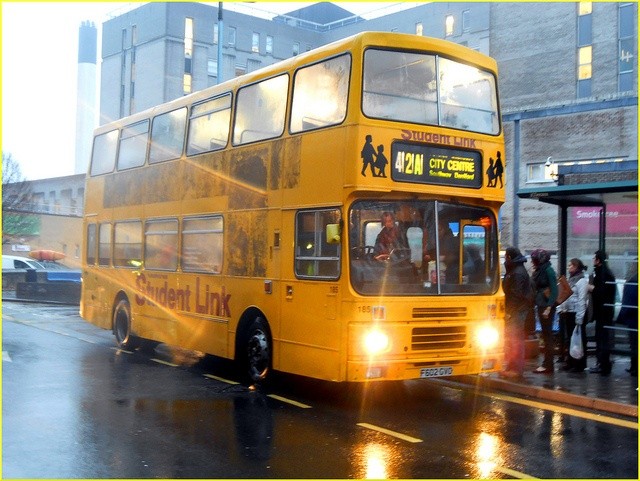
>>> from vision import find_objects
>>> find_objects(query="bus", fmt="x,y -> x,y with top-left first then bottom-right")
78,30 -> 505,383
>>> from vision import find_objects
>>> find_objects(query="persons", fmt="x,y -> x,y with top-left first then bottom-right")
587,250 -> 618,378
614,258 -> 639,378
424,217 -> 468,286
486,150 -> 504,190
557,260 -> 591,376
359,134 -> 389,179
361,253 -> 391,267
530,250 -> 560,374
498,244 -> 531,381
370,213 -> 414,265
466,244 -> 486,283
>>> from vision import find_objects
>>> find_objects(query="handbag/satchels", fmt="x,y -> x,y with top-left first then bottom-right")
568,325 -> 584,360
544,273 -> 573,307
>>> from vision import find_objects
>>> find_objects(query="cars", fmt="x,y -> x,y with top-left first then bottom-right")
2,256 -> 44,271
